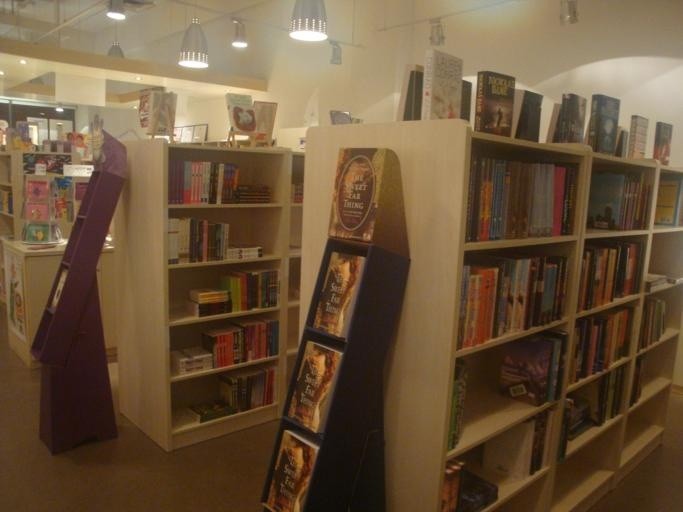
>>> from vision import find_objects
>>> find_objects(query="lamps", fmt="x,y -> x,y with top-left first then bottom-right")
107,23 -> 124,58
106,0 -> 126,20
177,17 -> 210,69
288,0 -> 329,42
231,20 -> 250,48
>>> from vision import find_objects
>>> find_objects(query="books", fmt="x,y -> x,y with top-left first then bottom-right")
420,49 -> 463,120
253,101 -> 278,140
473,71 -> 516,138
395,63 -> 425,120
565,366 -> 624,442
286,339 -> 344,437
569,305 -> 636,384
645,272 -> 683,293
402,70 -> 472,122
457,247 -> 568,348
447,359 -> 468,454
310,250 -> 367,341
654,173 -> 682,230
440,459 -> 499,511
264,429 -> 320,512
481,409 -> 558,480
558,93 -> 587,142
50,268 -> 68,308
185,362 -> 280,424
168,217 -> 263,264
627,115 -> 649,159
464,153 -> 580,242
183,265 -> 281,317
638,297 -> 666,353
631,354 -> 649,407
166,316 -> 279,375
137,86 -> 164,127
0,189 -> 12,215
225,93 -> 256,133
291,173 -> 303,204
588,166 -> 650,231
546,101 -> 562,145
588,94 -> 620,153
145,90 -> 177,136
510,88 -> 543,143
168,159 -> 273,204
653,121 -> 672,166
577,236 -> 644,314
494,329 -> 568,407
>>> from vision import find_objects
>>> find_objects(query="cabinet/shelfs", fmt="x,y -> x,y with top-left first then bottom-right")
0,150 -> 83,240
3,239 -> 116,371
300,119 -> 683,512
113,139 -> 306,452
